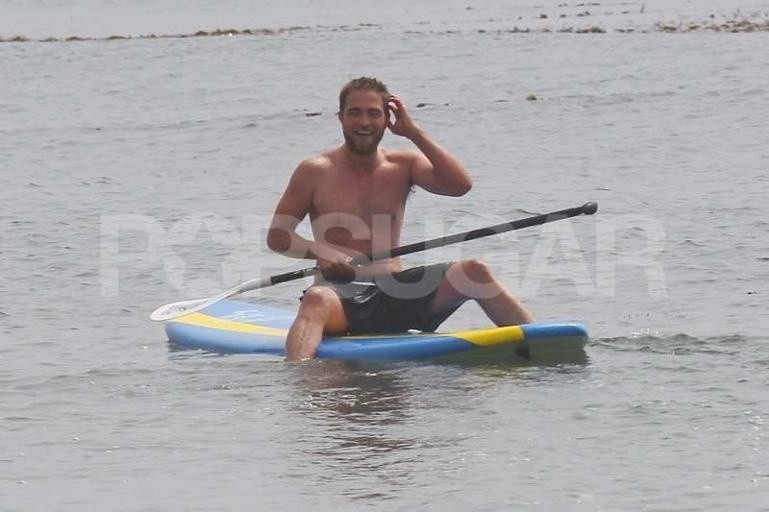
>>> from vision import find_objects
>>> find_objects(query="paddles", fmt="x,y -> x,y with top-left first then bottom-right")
148,202 -> 599,322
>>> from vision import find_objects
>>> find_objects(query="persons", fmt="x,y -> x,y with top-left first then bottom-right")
265,75 -> 537,363
